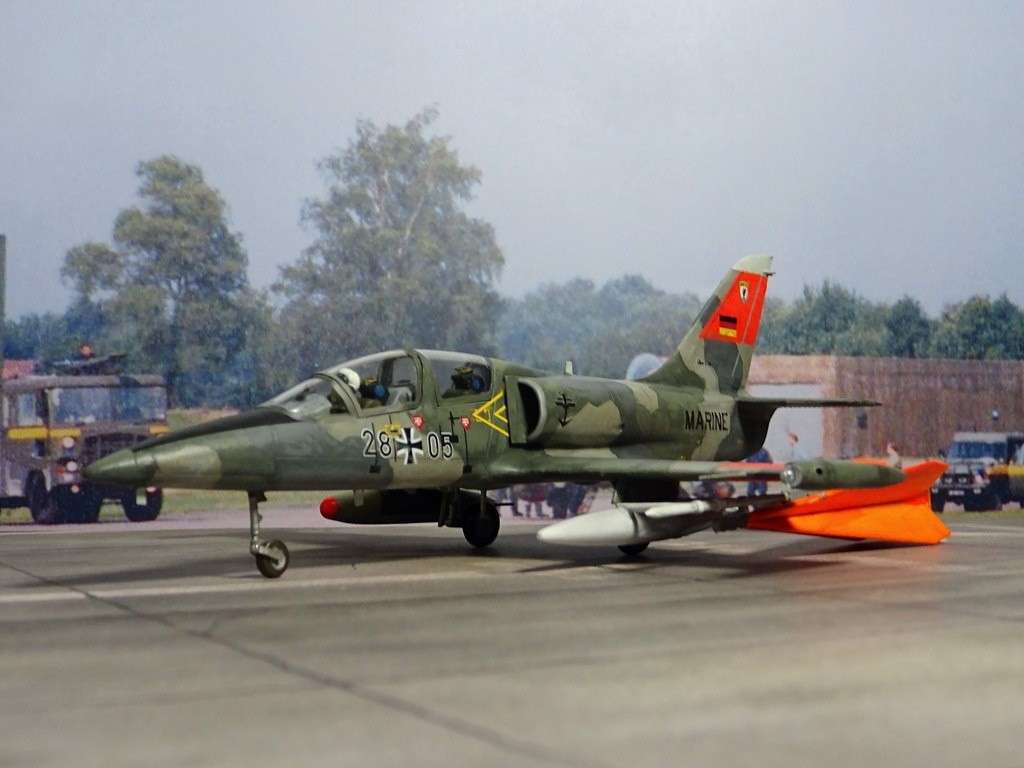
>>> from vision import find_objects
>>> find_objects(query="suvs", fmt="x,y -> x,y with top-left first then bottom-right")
931,432 -> 1024,512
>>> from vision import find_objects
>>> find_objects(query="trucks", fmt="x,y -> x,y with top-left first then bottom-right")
0,353 -> 170,526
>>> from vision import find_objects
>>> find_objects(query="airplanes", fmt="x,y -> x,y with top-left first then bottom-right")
79,252 -> 953,579
